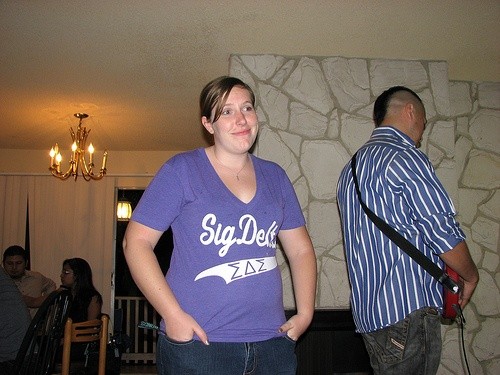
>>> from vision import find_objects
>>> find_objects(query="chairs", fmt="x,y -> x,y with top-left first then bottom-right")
9,289 -> 108,375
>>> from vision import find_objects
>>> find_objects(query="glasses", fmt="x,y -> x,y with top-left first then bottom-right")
62,270 -> 74,277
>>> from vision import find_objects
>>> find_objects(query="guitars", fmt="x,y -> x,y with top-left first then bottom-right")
441,264 -> 468,324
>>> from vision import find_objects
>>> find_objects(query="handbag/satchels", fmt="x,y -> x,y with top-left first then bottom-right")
85,313 -> 124,375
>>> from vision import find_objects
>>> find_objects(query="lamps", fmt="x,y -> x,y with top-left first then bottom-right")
50,113 -> 108,182
117,192 -> 132,221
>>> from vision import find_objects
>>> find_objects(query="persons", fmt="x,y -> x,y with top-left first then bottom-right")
336,86 -> 479,375
122,76 -> 317,375
0,266 -> 31,375
24,258 -> 102,363
3,246 -> 55,335
